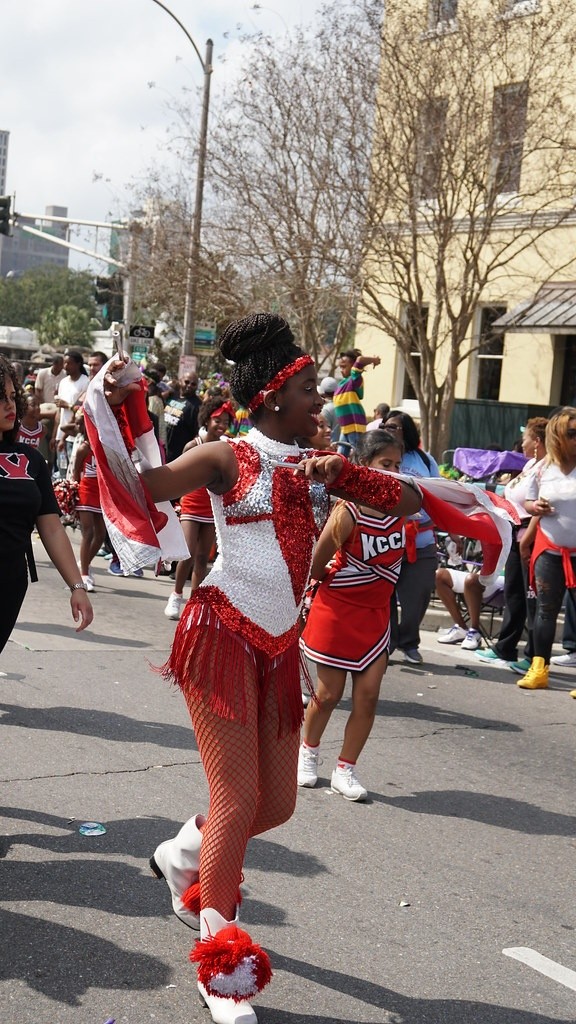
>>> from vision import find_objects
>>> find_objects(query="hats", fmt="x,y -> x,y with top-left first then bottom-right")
320,377 -> 338,392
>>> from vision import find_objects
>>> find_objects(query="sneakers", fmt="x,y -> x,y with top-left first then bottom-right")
571,689 -> 576,697
108,561 -> 124,575
476,648 -> 509,664
297,745 -> 323,788
331,770 -> 367,801
551,651 -> 576,667
133,569 -> 143,577
461,627 -> 482,650
510,657 -> 531,675
438,624 -> 468,643
517,657 -> 550,688
165,592 -> 183,620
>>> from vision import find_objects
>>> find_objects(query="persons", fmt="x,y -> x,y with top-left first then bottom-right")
297,348 -> 439,664
435,406 -> 576,699
11,351 -> 253,620
83,314 -> 422,1024
297,430 -> 406,801
0,352 -> 93,654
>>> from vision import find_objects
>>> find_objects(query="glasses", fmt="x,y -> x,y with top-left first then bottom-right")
379,423 -> 402,433
185,380 -> 196,386
567,428 -> 576,438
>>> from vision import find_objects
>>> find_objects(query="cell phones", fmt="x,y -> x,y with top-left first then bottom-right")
540,497 -> 550,508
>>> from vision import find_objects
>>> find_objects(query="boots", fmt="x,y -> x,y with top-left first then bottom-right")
150,814 -> 206,931
197,905 -> 257,1024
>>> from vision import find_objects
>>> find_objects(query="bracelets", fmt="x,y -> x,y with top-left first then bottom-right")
70,584 -> 88,593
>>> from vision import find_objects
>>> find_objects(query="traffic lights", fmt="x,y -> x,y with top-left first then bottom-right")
94,273 -> 123,324
0,195 -> 11,235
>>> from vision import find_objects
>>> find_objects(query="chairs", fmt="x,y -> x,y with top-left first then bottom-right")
458,588 -> 506,649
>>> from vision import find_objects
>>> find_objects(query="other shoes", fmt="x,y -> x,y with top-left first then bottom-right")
169,572 -> 176,578
405,648 -> 422,663
96,549 -> 113,560
77,561 -> 94,584
81,576 -> 93,592
